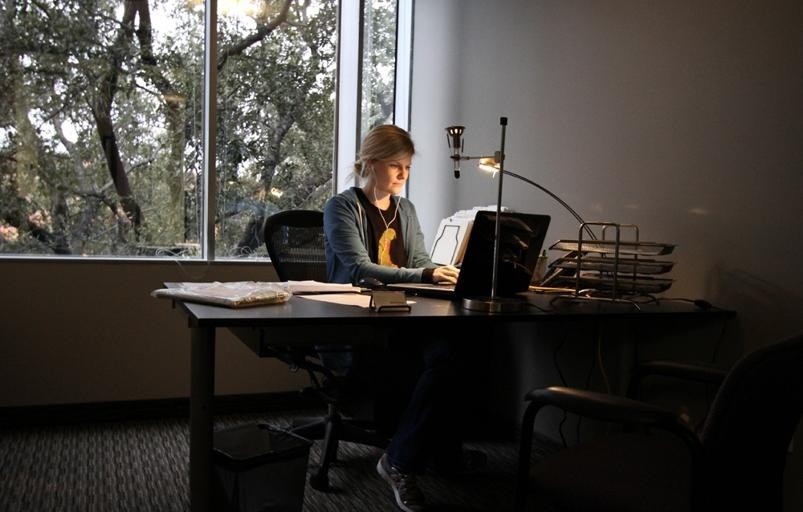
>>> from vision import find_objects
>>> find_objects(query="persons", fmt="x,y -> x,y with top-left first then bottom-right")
323,124 -> 461,511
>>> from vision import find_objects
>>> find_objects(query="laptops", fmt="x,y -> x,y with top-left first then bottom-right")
386,210 -> 550,301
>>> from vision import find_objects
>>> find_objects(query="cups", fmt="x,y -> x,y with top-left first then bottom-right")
529,255 -> 548,287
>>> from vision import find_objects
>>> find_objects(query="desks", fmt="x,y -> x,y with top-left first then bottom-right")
158,274 -> 740,512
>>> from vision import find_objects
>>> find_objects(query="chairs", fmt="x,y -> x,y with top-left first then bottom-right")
509,325 -> 801,510
263,209 -> 396,491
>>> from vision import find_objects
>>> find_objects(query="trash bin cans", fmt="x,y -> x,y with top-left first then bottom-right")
210,423 -> 314,512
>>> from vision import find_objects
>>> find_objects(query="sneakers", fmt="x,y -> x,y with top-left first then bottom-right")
376,452 -> 428,512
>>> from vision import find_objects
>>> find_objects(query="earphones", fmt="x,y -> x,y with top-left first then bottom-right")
369,164 -> 376,173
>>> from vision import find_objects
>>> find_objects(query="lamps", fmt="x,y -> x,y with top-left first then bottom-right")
442,112 -> 511,315
475,146 -> 614,274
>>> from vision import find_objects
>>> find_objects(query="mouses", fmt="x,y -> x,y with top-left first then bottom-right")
358,275 -> 384,288
